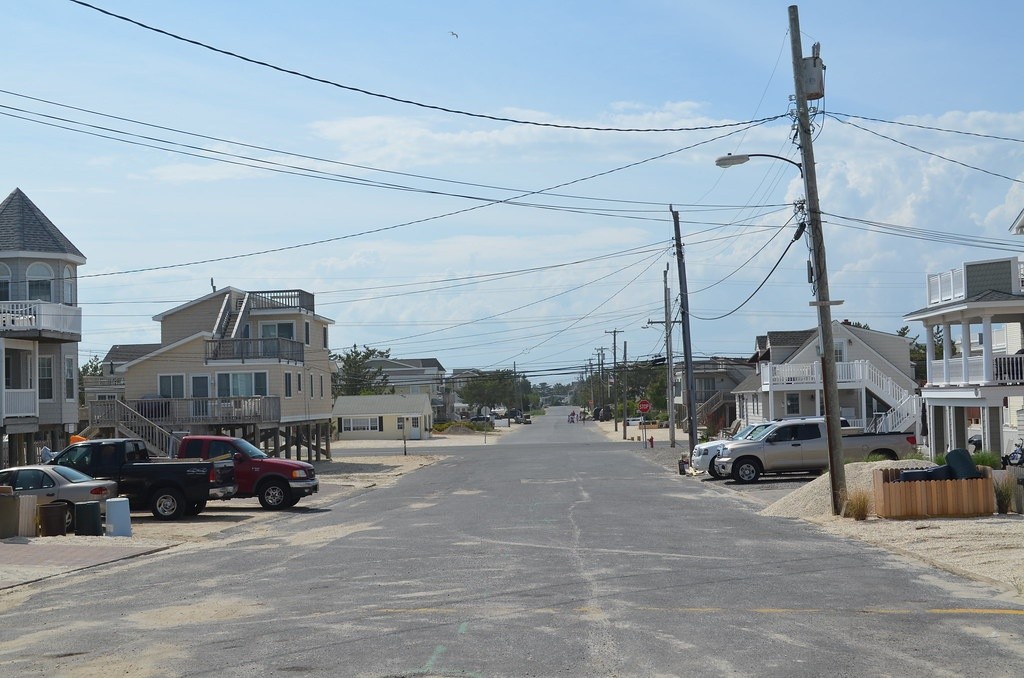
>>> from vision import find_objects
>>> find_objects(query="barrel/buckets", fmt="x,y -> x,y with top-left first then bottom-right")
75,500 -> 104,536
105,497 -> 132,536
36,502 -> 68,537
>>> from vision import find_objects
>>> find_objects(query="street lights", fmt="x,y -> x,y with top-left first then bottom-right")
714,152 -> 851,518
640,324 -> 676,448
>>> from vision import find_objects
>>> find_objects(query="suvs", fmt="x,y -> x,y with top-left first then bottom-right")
470,415 -> 495,430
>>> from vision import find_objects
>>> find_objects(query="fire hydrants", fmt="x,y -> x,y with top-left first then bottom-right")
647,436 -> 655,449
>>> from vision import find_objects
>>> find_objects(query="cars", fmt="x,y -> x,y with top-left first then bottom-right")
0,465 -> 118,532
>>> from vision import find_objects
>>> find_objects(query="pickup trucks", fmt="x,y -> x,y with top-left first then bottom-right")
149,435 -> 320,512
691,417 -> 866,481
714,419 -> 918,486
44,438 -> 217,521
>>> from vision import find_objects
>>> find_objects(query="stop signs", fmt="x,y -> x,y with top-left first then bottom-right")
637,400 -> 650,413
